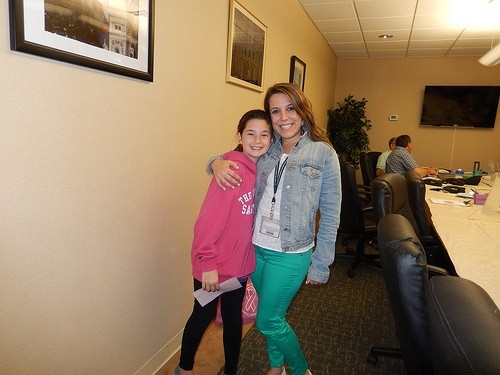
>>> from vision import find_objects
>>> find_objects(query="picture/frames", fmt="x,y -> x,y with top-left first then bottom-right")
8,0 -> 155,83
224,0 -> 268,92
290,55 -> 306,93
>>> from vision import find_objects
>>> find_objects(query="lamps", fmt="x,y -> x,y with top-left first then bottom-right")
477,32 -> 500,68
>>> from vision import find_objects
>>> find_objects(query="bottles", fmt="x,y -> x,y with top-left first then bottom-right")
454,168 -> 464,179
472,161 -> 480,174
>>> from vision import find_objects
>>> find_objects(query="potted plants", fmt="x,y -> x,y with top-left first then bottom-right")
327,94 -> 372,235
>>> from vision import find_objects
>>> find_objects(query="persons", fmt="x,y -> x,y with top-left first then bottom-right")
376,137 -> 398,176
385,135 -> 436,177
174,110 -> 271,375
206,82 -> 342,375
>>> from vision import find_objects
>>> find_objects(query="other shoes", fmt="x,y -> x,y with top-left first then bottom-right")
280,366 -> 286,375
307,369 -> 313,375
175,365 -> 194,375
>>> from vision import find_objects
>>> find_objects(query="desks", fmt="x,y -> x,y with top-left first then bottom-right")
424,174 -> 499,311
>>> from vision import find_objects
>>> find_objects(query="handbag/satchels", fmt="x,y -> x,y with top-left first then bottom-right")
216,276 -> 257,326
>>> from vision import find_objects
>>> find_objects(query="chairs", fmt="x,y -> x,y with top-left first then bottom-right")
335,150 -> 500,375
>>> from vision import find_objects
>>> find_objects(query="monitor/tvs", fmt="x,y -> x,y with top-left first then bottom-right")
419,85 -> 500,128
